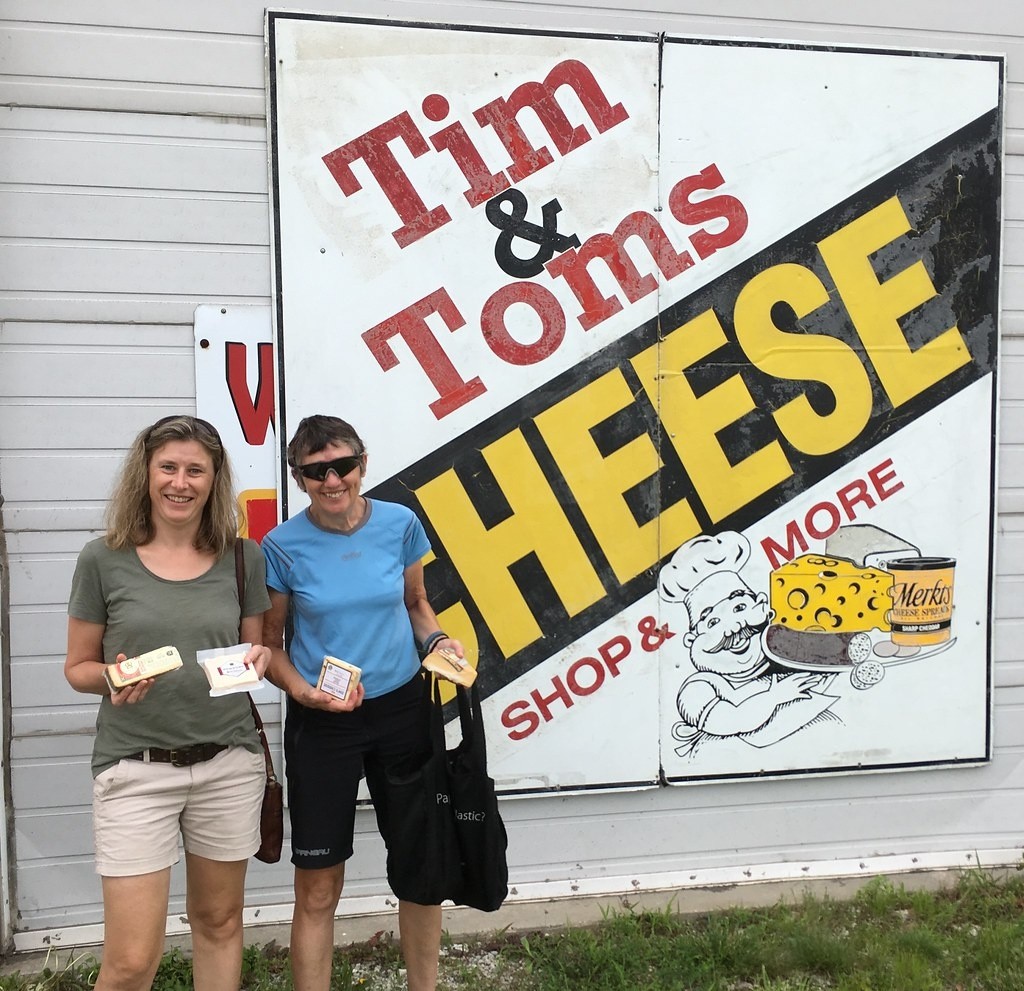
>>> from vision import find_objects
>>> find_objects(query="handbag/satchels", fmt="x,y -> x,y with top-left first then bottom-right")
253,781 -> 283,863
430,638 -> 508,912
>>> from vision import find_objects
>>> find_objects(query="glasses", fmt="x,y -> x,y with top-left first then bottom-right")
296,455 -> 361,481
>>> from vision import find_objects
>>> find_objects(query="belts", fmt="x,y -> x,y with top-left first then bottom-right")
124,743 -> 229,769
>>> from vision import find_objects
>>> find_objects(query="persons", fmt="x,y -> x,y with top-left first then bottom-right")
259,415 -> 464,991
65,413 -> 270,991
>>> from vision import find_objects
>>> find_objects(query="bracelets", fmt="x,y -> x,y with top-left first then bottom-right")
420,630 -> 449,654
